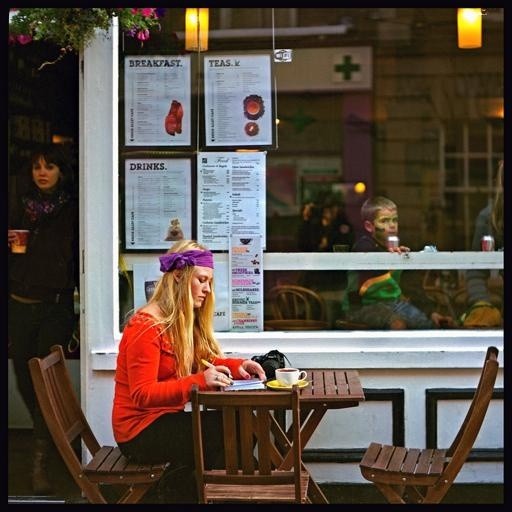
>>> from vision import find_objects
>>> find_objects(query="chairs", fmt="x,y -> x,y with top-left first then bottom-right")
263,236 -> 504,330
189,382 -> 312,506
356,344 -> 501,504
28,342 -> 174,504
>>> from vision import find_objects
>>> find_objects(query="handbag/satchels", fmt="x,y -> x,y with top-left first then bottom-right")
465,302 -> 503,326
251,350 -> 285,380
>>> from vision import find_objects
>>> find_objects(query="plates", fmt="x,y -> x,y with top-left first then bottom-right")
266,380 -> 309,392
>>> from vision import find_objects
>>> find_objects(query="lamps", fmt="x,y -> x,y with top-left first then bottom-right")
454,6 -> 485,52
182,8 -> 212,54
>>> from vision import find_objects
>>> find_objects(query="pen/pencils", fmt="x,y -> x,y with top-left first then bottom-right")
201,358 -> 234,379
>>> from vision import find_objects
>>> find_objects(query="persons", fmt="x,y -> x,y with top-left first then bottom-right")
7,143 -> 78,496
465,162 -> 504,326
112,239 -> 267,505
342,196 -> 455,329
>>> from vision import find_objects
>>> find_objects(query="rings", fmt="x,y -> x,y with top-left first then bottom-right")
216,374 -> 219,380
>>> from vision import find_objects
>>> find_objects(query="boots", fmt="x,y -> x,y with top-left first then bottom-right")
30,436 -> 53,495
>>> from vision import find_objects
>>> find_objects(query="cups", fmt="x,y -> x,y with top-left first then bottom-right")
274,367 -> 309,386
10,229 -> 31,255
480,235 -> 495,252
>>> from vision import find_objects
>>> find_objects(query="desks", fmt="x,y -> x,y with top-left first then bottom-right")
196,366 -> 368,503
